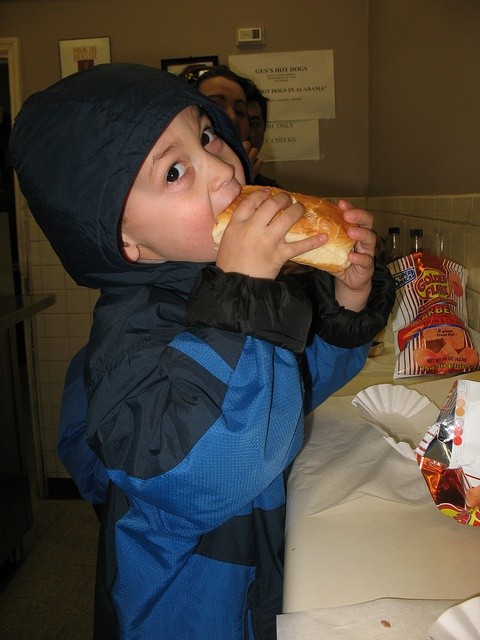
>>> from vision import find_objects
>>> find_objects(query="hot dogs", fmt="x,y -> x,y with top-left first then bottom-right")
213,183 -> 356,277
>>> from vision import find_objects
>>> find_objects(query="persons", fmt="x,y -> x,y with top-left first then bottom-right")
14,63 -> 397,640
236,78 -> 283,192
180,62 -> 262,186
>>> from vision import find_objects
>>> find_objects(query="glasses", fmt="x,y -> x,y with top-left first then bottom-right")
182,65 -> 219,90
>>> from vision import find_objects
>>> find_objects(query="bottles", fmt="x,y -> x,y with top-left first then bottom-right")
410,228 -> 423,255
380,227 -> 402,268
